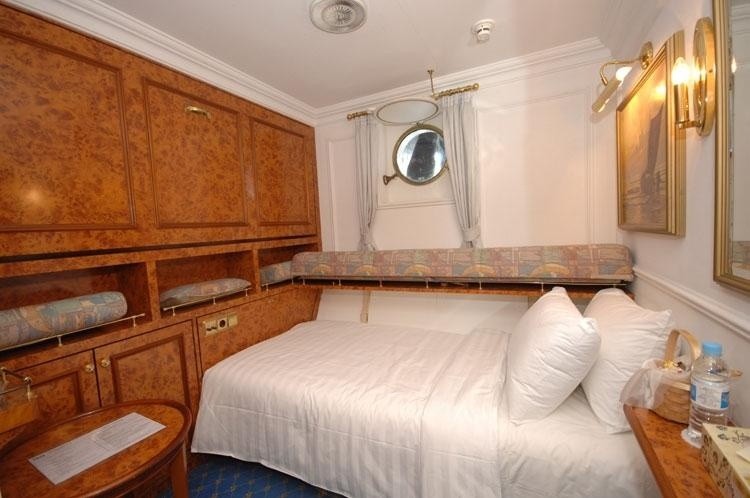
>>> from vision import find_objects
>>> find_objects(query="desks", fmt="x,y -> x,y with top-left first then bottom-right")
623,398 -> 750,498
0,399 -> 193,498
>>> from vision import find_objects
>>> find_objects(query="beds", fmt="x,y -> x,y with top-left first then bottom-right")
191,287 -> 673,498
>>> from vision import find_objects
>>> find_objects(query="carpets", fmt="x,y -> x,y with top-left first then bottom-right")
188,453 -> 348,498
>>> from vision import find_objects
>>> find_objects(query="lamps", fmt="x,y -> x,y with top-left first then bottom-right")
591,41 -> 653,114
671,17 -> 716,136
310,0 -> 368,34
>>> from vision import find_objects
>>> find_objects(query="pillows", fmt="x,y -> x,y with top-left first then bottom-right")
507,286 -> 600,421
261,261 -> 292,285
292,243 -> 636,283
583,285 -> 673,432
0,291 -> 128,349
160,278 -> 252,307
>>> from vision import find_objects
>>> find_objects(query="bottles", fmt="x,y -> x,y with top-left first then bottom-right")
688,342 -> 731,446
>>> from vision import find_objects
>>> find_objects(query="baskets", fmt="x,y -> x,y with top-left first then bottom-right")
645,329 -> 737,428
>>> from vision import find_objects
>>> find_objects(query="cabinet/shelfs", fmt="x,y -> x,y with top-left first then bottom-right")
0,321 -> 199,446
251,101 -> 321,241
1,0 -> 145,259
133,58 -> 255,250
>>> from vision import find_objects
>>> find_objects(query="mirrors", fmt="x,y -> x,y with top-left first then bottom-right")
713,0 -> 750,295
375,98 -> 450,187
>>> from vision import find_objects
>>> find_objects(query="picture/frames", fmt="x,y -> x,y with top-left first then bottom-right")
616,32 -> 685,238
